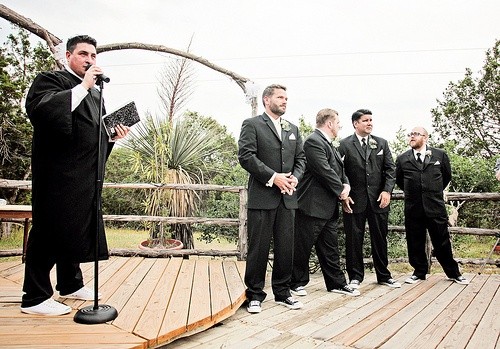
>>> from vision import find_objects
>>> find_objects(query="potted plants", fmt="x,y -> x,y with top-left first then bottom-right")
135,121 -> 184,251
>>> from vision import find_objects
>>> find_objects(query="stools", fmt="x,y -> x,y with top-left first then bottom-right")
0,204 -> 33,262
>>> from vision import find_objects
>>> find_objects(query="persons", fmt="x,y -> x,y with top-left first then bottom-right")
19,34 -> 130,318
288,108 -> 360,299
392,126 -> 471,286
232,84 -> 303,314
335,109 -> 403,291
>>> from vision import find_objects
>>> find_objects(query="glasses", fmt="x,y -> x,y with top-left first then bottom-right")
410,132 -> 426,137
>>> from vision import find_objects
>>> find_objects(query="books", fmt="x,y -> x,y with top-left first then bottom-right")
101,100 -> 141,139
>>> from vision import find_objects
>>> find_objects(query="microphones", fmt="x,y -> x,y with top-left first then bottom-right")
86,64 -> 110,83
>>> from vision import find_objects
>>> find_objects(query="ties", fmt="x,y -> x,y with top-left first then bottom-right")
362,138 -> 367,152
417,153 -> 423,164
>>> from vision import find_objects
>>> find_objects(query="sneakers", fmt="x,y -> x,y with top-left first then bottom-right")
450,275 -> 469,284
331,283 -> 360,297
20,298 -> 72,315
290,286 -> 307,296
350,279 -> 361,289
276,295 -> 301,310
405,275 -> 420,284
59,287 -> 101,300
380,279 -> 400,288
248,298 -> 261,313
